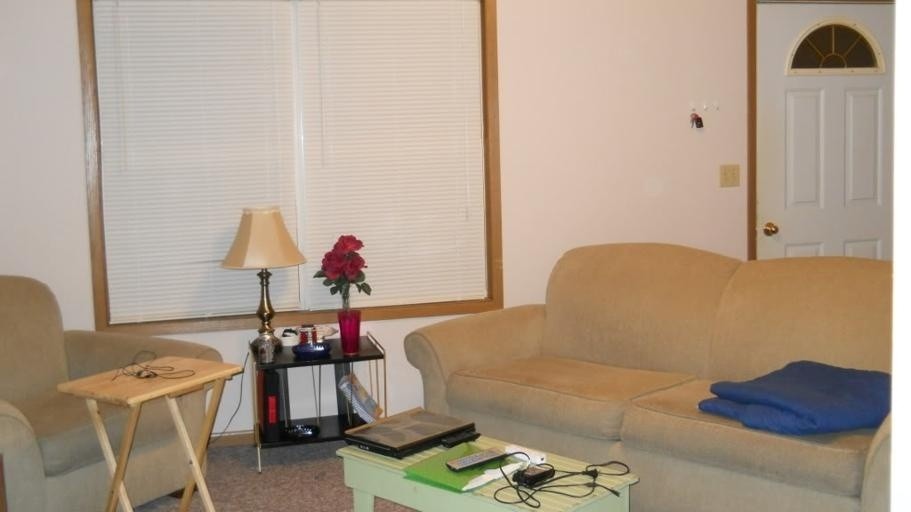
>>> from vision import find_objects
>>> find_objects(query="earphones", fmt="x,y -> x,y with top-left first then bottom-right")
586,468 -> 599,479
135,370 -> 143,375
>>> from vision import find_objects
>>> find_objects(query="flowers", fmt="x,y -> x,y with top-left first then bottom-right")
312,235 -> 372,309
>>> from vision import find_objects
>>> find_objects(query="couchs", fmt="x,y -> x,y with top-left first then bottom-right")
403,242 -> 891,512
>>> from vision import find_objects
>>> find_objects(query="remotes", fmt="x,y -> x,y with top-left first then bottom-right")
446,448 -> 503,471
441,431 -> 483,447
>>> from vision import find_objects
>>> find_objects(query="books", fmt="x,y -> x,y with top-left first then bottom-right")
339,374 -> 382,423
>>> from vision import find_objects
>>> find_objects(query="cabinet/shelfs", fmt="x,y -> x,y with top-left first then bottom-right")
248,330 -> 387,473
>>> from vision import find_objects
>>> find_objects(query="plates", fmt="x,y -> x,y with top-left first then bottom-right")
292,343 -> 330,357
279,424 -> 321,438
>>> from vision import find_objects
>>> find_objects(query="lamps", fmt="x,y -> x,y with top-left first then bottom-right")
219,206 -> 307,353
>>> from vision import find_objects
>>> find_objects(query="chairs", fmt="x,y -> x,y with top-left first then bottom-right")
0,274 -> 225,512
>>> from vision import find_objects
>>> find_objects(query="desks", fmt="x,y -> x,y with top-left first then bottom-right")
56,354 -> 244,512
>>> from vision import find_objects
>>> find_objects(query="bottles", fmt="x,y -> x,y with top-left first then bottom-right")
299,324 -> 317,343
